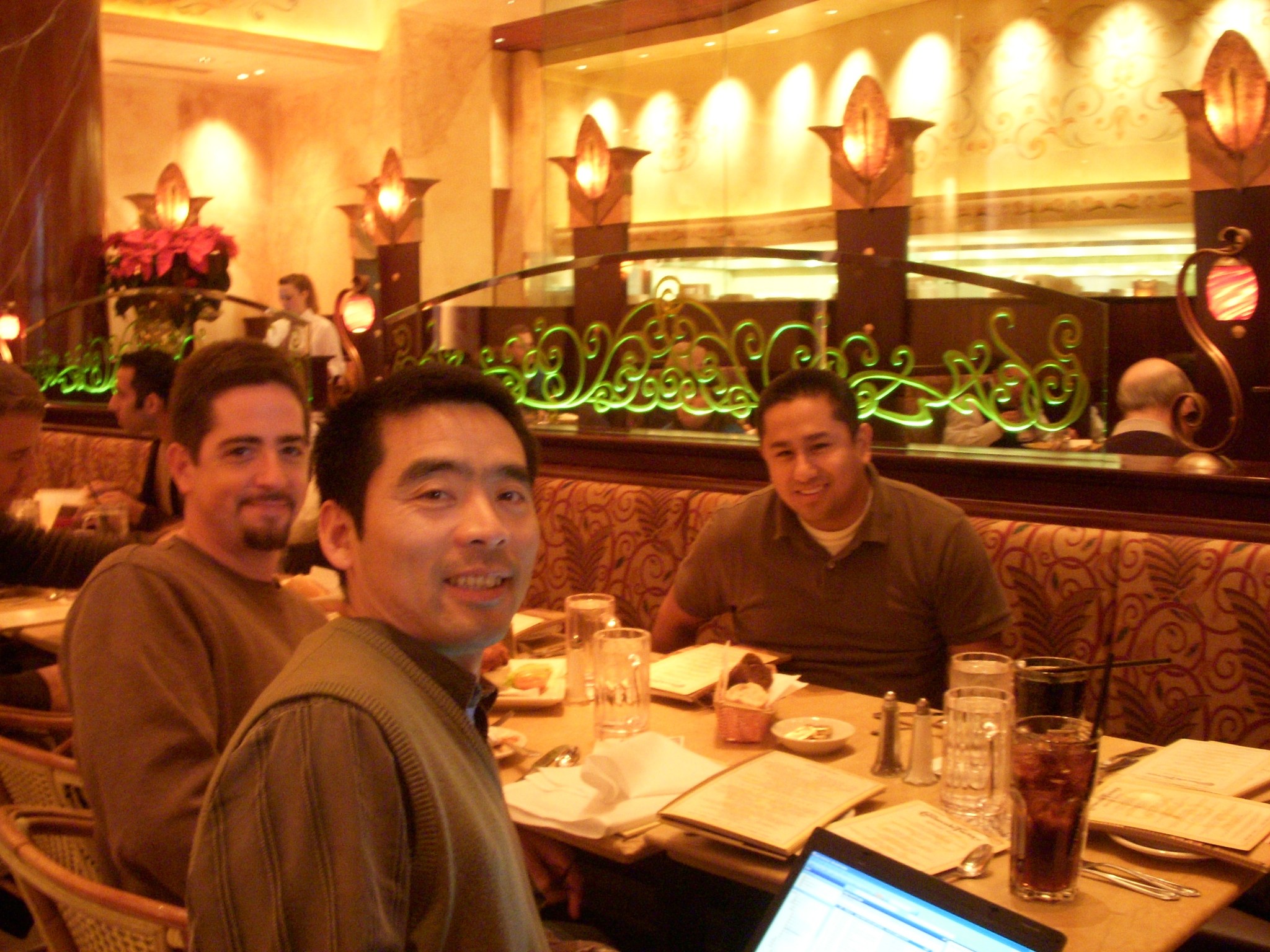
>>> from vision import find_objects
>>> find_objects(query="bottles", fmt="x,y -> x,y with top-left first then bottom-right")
869,690 -> 904,779
900,697 -> 939,786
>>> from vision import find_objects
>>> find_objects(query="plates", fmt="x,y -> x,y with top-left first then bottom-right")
1110,832 -> 1214,863
487,726 -> 527,760
483,655 -> 566,711
770,716 -> 857,755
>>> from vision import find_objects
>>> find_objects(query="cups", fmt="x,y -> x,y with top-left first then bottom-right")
564,592 -> 616,703
1009,655 -> 1091,719
10,498 -> 42,526
590,626 -> 653,735
96,511 -> 130,537
1008,714 -> 1103,903
948,650 -> 1017,692
940,686 -> 1017,817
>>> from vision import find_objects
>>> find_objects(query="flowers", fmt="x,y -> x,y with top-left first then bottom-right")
98,220 -> 241,314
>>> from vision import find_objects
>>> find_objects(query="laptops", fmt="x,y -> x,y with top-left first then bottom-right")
744,827 -> 1067,952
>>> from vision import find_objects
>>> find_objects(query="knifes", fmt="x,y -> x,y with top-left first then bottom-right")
515,742 -> 570,783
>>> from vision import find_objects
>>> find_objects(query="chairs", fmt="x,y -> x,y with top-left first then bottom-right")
0,733 -> 91,811
0,639 -> 77,757
0,803 -> 189,952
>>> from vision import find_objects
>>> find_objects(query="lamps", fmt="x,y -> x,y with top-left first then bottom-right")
807,76 -> 937,212
546,114 -> 652,228
1162,30 -> 1270,191
1170,226 -> 1257,450
358,149 -> 441,246
333,272 -> 376,390
123,163 -> 215,235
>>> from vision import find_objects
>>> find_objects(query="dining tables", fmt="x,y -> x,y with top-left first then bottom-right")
0,584 -> 1267,952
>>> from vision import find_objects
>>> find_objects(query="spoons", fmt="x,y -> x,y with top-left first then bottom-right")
554,745 -> 580,767
938,844 -> 993,882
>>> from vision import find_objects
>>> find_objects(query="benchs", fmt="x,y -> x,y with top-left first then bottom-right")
19,426 -> 1270,750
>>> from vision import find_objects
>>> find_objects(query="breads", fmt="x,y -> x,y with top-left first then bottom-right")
724,652 -> 773,710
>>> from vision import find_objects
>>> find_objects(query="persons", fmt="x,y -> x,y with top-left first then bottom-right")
80,346 -> 185,533
652,369 -> 1013,711
0,362 -> 185,712
495,324 -> 553,400
645,339 -> 747,433
942,358 -> 1077,448
59,339 -> 619,952
263,274 -> 346,377
1099,358 -> 1203,458
